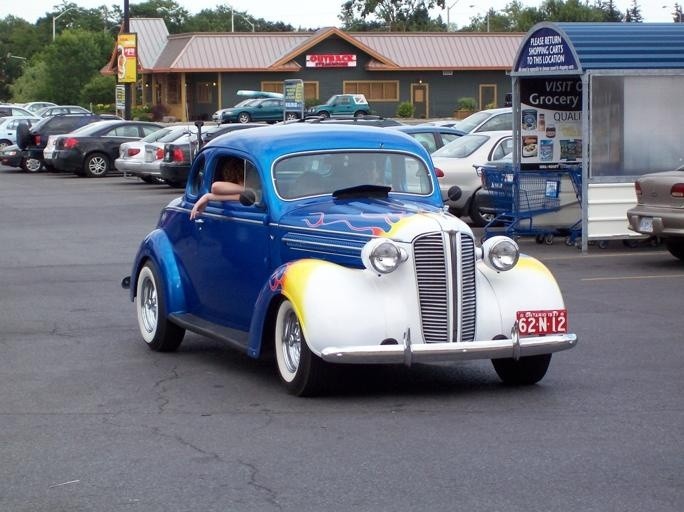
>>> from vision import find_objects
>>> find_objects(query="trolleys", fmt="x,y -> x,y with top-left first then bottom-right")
472,162 -> 569,246
564,163 -> 662,249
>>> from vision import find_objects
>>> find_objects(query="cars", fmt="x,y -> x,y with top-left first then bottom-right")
626,162 -> 684,262
266,116 -> 406,129
306,94 -> 370,119
0,102 -> 221,188
221,99 -> 306,124
450,106 -> 518,134
390,130 -> 519,227
159,125 -> 270,182
211,90 -> 282,125
414,121 -> 458,128
120,123 -> 577,397
383,126 -> 468,156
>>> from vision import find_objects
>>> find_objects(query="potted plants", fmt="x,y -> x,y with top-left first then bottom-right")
453,94 -> 477,119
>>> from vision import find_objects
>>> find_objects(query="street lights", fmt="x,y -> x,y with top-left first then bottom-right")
231,13 -> 254,33
52,7 -> 78,43
469,5 -> 489,33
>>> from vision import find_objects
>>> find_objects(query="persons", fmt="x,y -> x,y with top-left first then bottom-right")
189,168 -> 260,220
